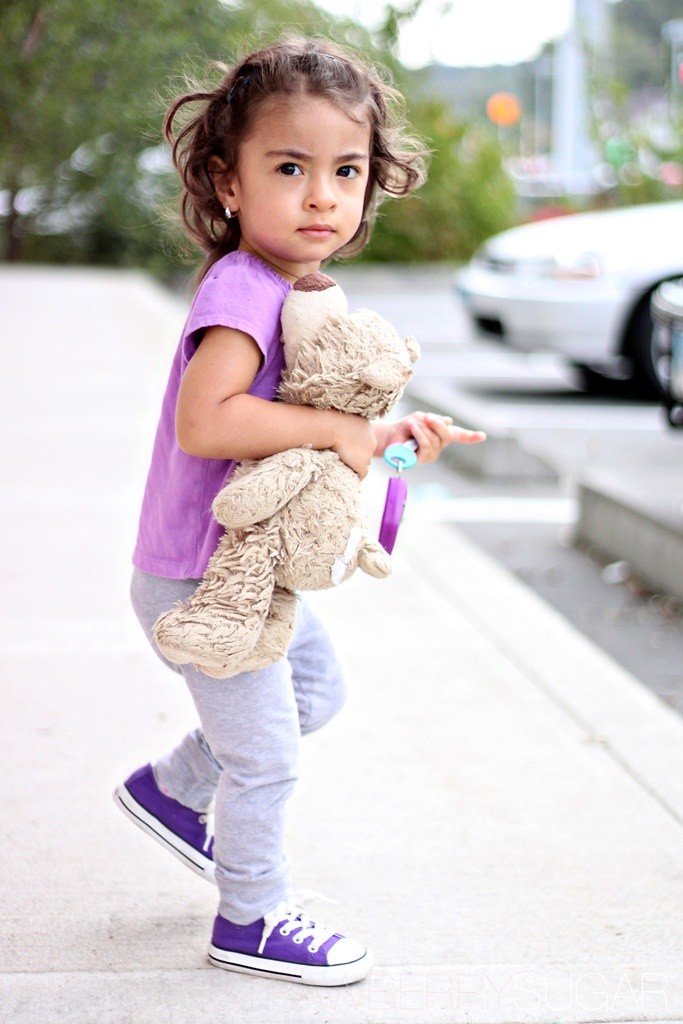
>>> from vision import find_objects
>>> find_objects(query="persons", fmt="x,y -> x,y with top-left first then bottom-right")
112,39 -> 486,988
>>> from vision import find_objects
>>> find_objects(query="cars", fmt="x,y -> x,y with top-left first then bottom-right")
455,199 -> 683,406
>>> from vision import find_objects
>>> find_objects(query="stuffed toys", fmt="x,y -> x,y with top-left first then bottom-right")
155,272 -> 420,679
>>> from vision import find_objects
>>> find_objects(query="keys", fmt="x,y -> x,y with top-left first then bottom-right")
383,439 -> 418,470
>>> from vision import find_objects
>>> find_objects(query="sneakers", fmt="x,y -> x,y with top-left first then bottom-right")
116,765 -> 218,873
207,912 -> 371,988
